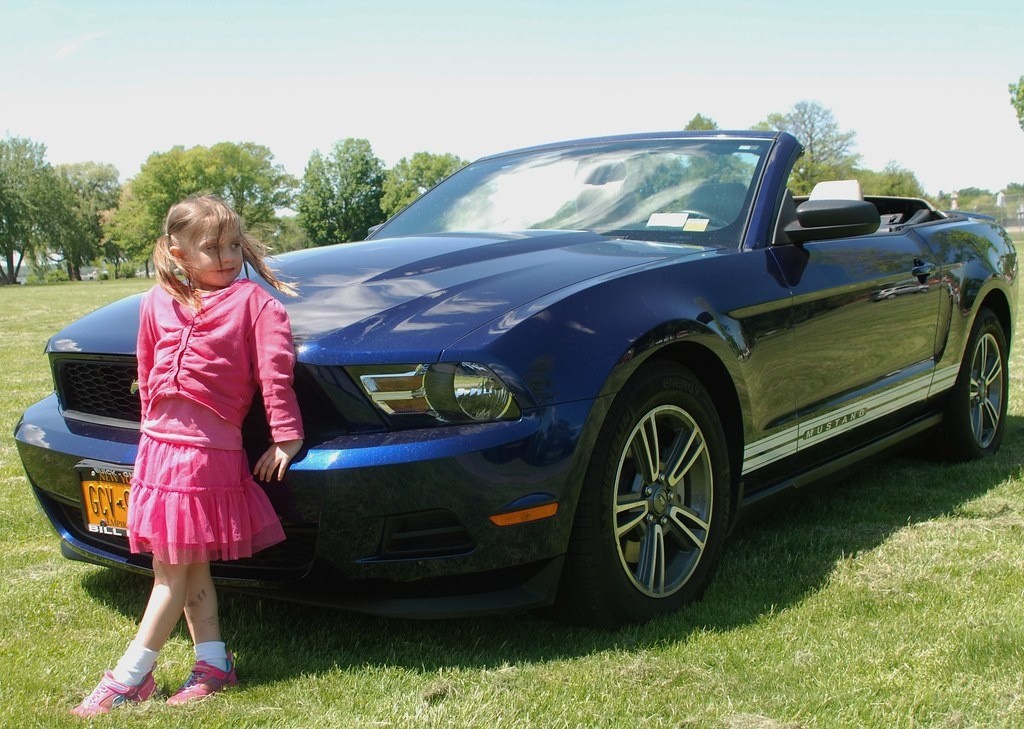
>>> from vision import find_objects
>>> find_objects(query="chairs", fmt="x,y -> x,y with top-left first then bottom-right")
686,182 -> 746,228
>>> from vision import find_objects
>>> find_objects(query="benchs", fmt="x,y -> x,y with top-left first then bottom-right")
808,178 -> 865,203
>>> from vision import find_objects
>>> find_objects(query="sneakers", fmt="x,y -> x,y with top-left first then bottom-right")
165,651 -> 238,708
70,660 -> 158,722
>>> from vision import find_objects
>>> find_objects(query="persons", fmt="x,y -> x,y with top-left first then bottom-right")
66,196 -> 304,715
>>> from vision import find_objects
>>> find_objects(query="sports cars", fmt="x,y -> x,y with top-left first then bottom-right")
14,129 -> 1020,624
